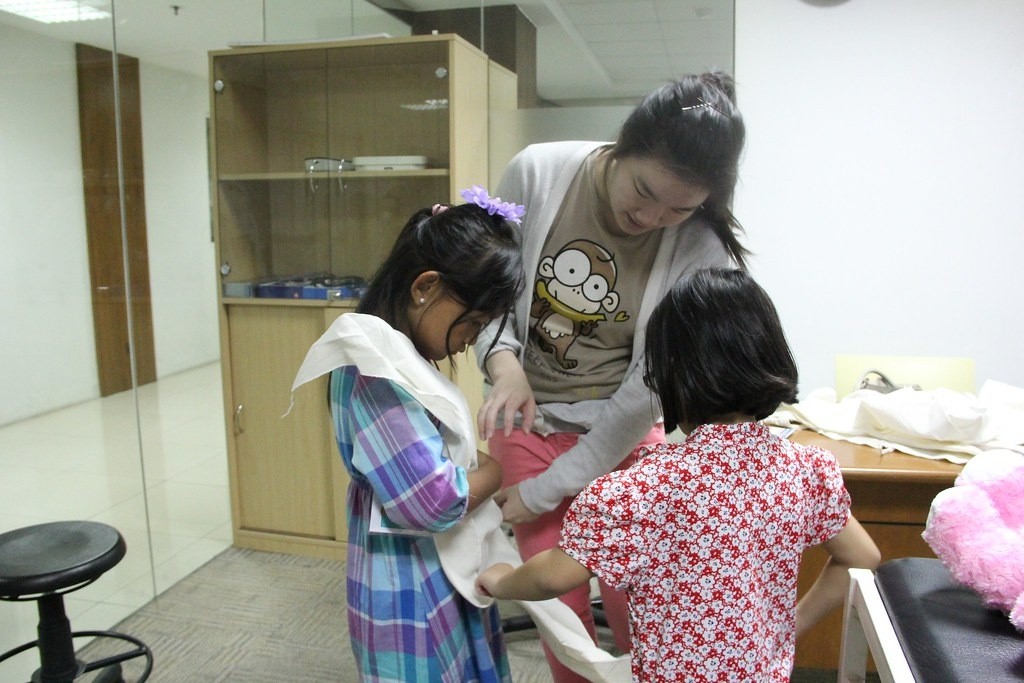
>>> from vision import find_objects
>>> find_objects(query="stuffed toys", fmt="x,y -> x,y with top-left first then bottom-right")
923,449 -> 1024,628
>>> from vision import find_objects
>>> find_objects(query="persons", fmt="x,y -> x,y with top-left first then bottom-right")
326,186 -> 527,683
476,268 -> 881,683
476,73 -> 747,683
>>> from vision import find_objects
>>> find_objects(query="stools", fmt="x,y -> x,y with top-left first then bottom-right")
0,521 -> 154,683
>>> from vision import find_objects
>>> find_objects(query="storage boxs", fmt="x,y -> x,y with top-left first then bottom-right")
352,156 -> 440,172
222,279 -> 370,300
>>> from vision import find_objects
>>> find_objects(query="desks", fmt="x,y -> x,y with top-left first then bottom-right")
789,427 -> 967,677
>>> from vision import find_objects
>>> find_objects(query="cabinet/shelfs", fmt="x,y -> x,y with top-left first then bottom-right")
207,32 -> 490,565
491,60 -> 519,202
